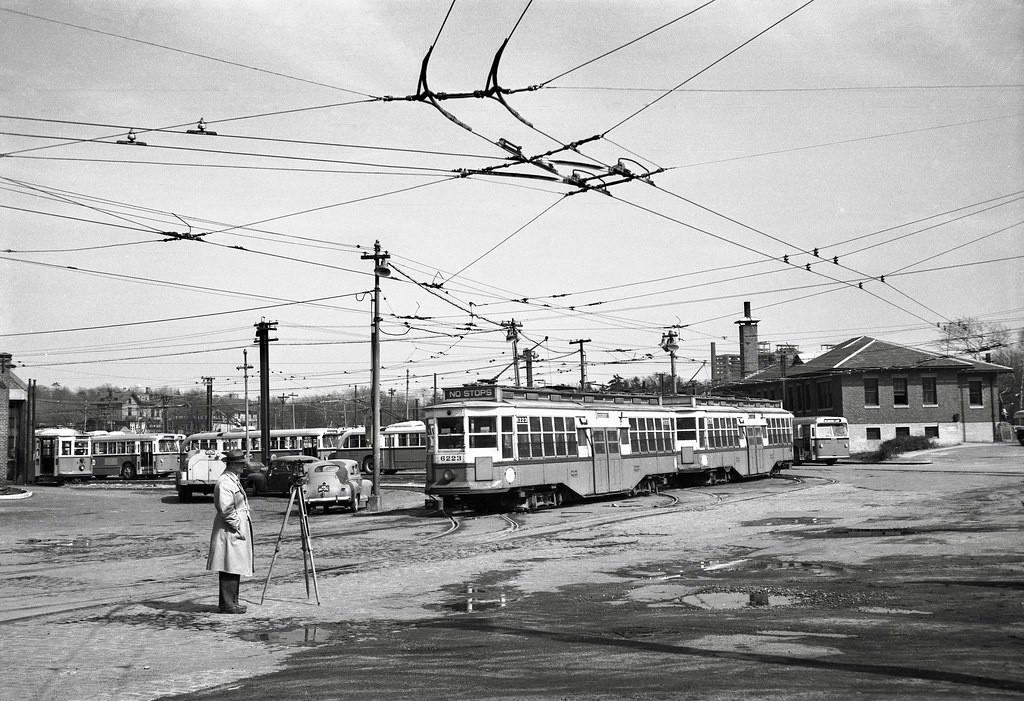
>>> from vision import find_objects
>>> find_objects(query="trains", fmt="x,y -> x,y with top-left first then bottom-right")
421,384 -> 795,512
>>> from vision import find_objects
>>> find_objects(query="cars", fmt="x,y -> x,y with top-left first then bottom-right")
247,455 -> 320,498
301,460 -> 373,511
178,450 -> 227,501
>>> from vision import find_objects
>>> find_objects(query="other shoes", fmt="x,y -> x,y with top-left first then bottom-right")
219,604 -> 248,614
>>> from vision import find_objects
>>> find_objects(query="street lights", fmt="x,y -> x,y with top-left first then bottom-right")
501,318 -> 523,386
361,240 -> 391,511
663,330 -> 679,393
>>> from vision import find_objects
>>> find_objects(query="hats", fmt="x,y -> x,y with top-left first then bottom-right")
221,449 -> 249,463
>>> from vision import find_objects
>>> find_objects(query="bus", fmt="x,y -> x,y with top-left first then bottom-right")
335,425 -> 427,474
32,425 -> 94,483
792,417 -> 850,466
180,427 -> 342,466
90,433 -> 186,480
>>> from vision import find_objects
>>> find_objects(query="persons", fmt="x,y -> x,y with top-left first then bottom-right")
447,423 -> 464,448
206,450 -> 255,613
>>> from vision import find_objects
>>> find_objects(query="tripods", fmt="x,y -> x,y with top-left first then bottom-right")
260,476 -> 322,607
